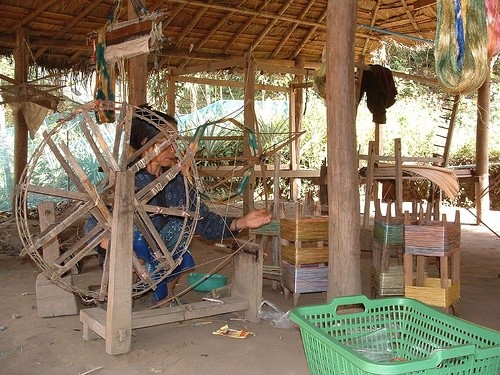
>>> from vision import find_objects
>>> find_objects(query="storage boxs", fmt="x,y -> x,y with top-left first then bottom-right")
290,294 -> 500,375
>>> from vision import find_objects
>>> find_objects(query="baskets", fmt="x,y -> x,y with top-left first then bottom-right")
288,294 -> 500,375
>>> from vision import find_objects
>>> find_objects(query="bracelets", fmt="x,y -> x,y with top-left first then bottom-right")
234,217 -> 243,233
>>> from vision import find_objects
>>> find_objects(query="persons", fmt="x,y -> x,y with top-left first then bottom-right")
84,103 -> 273,311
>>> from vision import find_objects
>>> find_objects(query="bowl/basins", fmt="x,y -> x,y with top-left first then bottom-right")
187,273 -> 228,292
